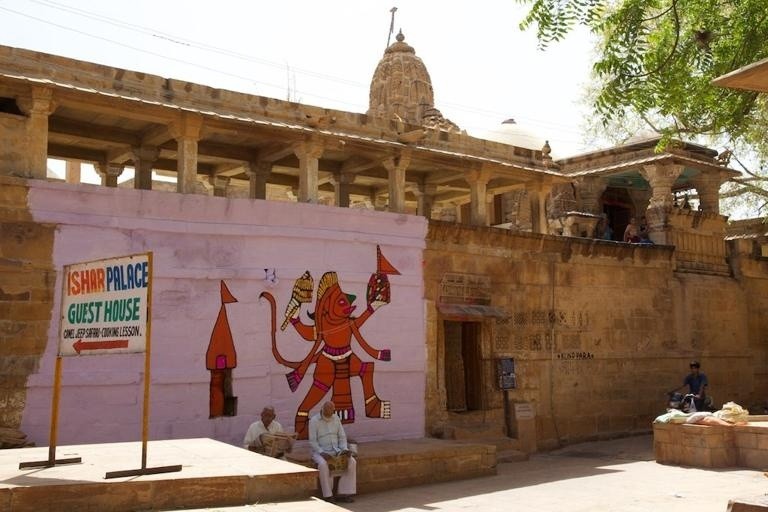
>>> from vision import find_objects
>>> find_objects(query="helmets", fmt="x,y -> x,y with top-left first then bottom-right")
689,360 -> 700,368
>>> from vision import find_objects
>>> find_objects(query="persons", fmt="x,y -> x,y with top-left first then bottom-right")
666,361 -> 707,412
637,214 -> 649,243
622,216 -> 639,242
307,400 -> 357,505
594,217 -> 612,240
240,405 -> 296,463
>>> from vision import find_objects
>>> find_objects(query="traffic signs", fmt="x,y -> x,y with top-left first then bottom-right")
58,251 -> 154,356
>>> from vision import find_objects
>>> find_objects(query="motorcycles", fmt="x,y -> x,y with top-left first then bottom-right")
666,391 -> 717,413
